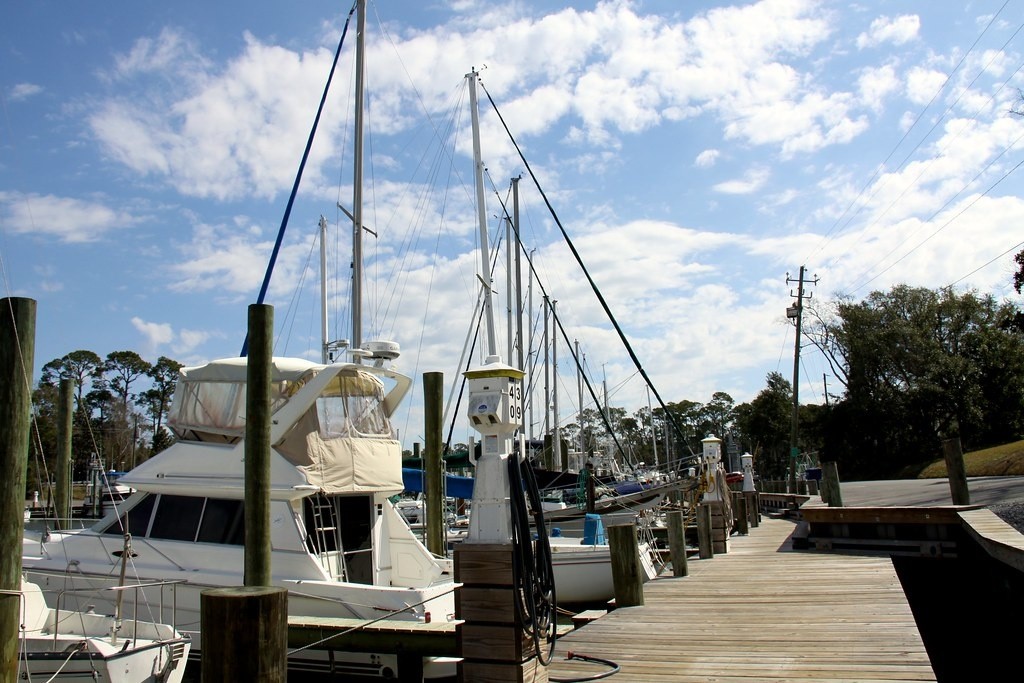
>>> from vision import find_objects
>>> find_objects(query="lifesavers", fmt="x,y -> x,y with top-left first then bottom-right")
598,493 -> 614,507
647,478 -> 655,485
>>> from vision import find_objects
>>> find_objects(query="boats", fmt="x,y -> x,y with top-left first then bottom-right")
81,469 -> 139,518
24,356 -> 456,682
395,457 -> 734,548
17,571 -> 198,683
418,522 -> 659,602
